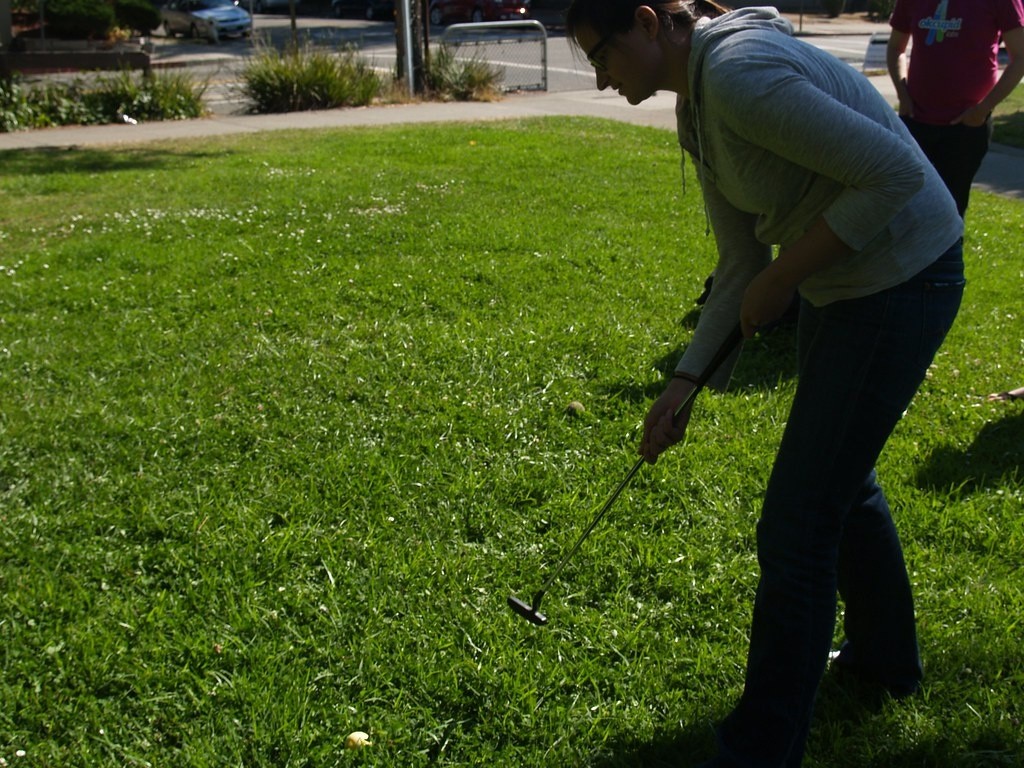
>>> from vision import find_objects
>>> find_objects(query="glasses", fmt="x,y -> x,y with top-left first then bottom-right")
587,4 -> 659,71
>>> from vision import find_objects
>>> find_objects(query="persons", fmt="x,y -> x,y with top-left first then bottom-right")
887,0 -> 1024,221
569,0 -> 964,740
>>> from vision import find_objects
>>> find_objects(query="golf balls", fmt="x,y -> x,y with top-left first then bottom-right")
344,731 -> 373,749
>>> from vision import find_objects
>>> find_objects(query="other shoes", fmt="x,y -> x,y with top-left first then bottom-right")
703,754 -> 742,768
824,650 -> 921,702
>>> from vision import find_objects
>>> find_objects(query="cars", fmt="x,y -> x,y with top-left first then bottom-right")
428,0 -> 534,31
160,0 -> 254,45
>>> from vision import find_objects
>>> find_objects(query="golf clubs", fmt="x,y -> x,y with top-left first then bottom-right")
506,324 -> 742,627
